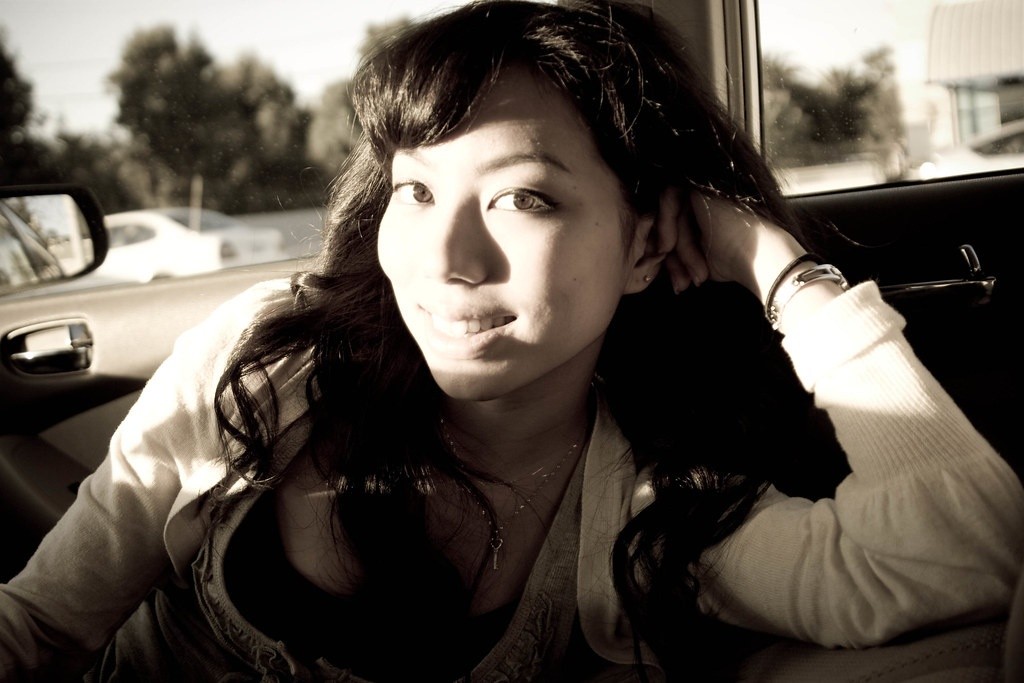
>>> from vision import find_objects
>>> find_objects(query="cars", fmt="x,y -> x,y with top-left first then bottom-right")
883,119 -> 1023,186
88,206 -> 284,283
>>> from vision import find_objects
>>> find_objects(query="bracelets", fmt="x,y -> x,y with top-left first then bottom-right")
764,254 -> 849,329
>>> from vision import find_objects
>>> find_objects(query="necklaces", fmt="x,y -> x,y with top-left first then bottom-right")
434,402 -> 594,569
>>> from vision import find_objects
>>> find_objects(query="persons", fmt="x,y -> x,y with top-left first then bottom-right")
0,0 -> 1024,683
